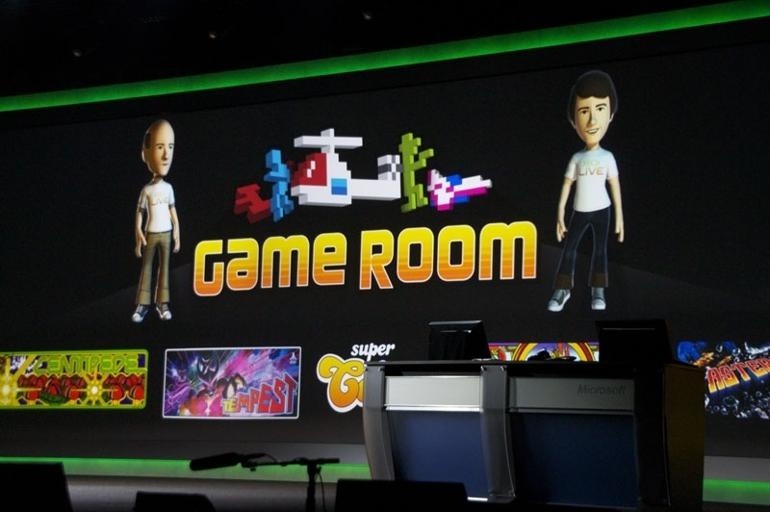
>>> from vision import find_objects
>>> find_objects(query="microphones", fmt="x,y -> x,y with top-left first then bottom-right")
190,452 -> 266,470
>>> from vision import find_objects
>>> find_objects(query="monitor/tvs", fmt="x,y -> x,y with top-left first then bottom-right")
595,319 -> 675,363
426,319 -> 491,360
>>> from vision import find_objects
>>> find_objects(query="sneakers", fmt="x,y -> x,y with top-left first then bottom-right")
590,287 -> 606,311
155,302 -> 173,321
547,289 -> 571,313
130,304 -> 150,323
